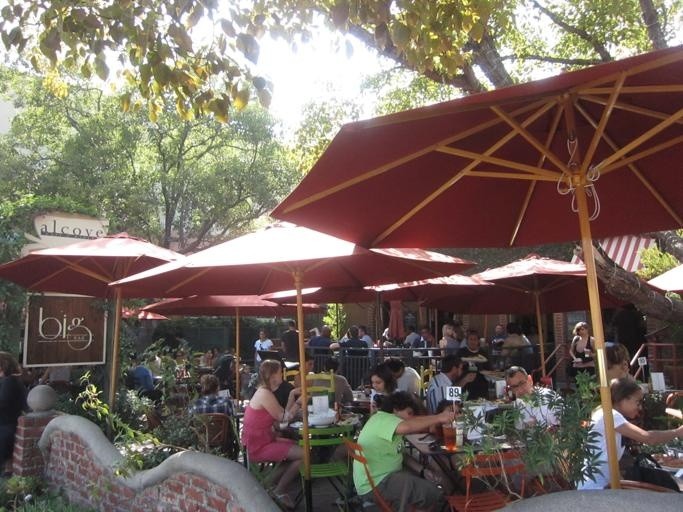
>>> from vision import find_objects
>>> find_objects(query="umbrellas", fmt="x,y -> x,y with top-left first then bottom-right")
0,230 -> 186,441
121,305 -> 171,322
646,262 -> 683,293
471,253 -> 664,388
106,221 -> 478,511
138,294 -> 329,412
268,42 -> 683,489
260,274 -> 497,363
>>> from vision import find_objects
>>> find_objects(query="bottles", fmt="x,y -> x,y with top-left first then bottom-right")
454,428 -> 464,446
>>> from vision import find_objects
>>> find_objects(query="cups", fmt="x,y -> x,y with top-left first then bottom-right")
584,348 -> 590,357
427,350 -> 433,359
441,424 -> 457,451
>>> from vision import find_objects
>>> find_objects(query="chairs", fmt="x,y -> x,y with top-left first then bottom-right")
138,340 -> 683,511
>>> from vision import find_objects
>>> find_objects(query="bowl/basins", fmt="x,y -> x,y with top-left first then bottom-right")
290,403 -> 335,428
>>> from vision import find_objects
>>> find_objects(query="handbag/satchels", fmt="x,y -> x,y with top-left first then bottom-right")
566,360 -> 577,375
623,452 -> 680,493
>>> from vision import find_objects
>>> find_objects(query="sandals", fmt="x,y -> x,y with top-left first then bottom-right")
271,489 -> 295,509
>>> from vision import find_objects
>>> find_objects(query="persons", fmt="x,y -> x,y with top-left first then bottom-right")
0,322 -> 683,512
